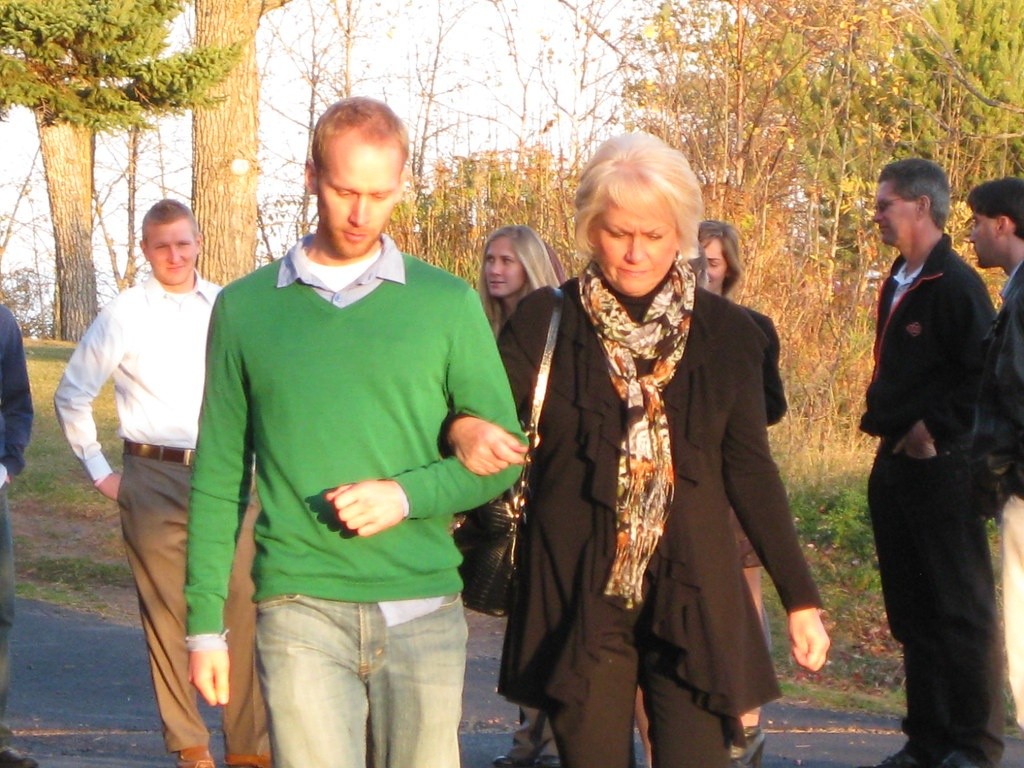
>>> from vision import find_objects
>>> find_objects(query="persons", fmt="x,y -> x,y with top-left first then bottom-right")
470,223 -> 566,342
0,301 -> 44,766
846,157 -> 1011,767
671,208 -> 831,768
184,103 -> 528,768
441,128 -> 832,768
950,176 -> 1023,749
50,199 -> 321,764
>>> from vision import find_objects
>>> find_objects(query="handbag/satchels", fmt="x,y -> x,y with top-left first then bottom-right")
446,478 -> 531,613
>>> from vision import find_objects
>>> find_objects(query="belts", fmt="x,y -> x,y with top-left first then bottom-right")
122,441 -> 195,467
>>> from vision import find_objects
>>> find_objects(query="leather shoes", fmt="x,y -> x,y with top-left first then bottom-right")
532,755 -> 561,768
490,754 -> 530,768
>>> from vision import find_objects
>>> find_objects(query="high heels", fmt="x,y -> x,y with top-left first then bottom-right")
729,725 -> 766,768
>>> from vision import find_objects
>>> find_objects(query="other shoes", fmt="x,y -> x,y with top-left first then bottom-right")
932,741 -> 996,767
177,746 -> 215,768
872,742 -> 928,767
223,753 -> 273,768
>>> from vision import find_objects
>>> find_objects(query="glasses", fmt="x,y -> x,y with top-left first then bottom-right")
874,197 -> 906,212
